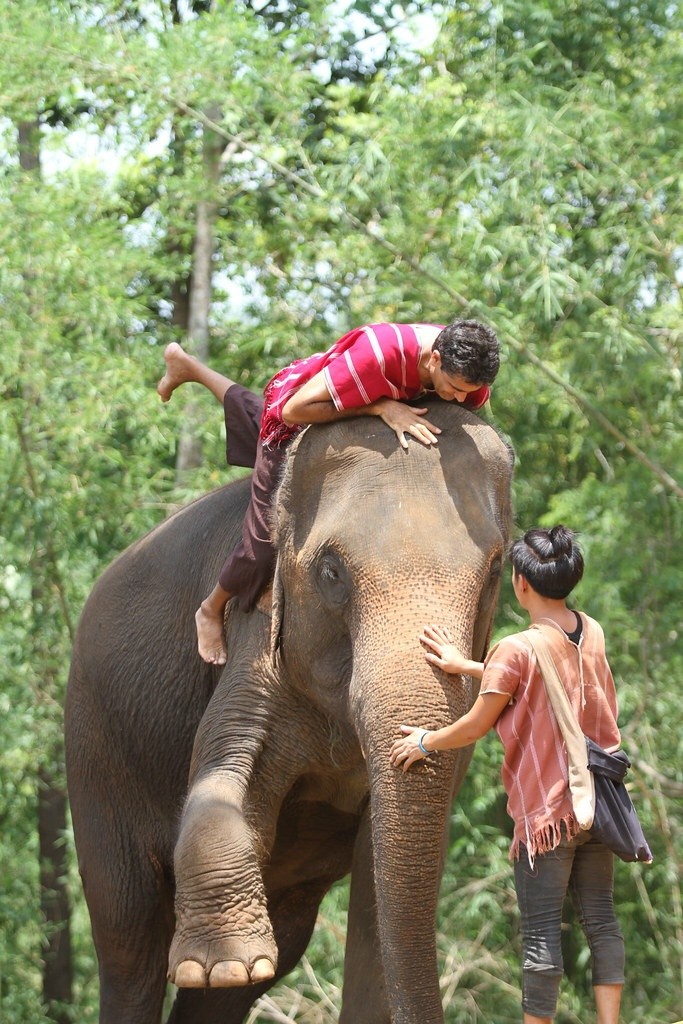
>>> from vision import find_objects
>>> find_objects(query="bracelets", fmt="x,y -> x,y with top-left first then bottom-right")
418,731 -> 435,755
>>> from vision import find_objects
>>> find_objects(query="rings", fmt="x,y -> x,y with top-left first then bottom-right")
449,639 -> 454,645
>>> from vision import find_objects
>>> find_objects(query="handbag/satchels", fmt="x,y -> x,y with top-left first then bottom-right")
565,735 -> 654,864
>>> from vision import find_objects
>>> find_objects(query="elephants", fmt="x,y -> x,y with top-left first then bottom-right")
62,395 -> 516,1024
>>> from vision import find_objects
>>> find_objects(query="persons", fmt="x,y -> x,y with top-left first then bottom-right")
156,320 -> 500,667
390,526 -> 627,1024
